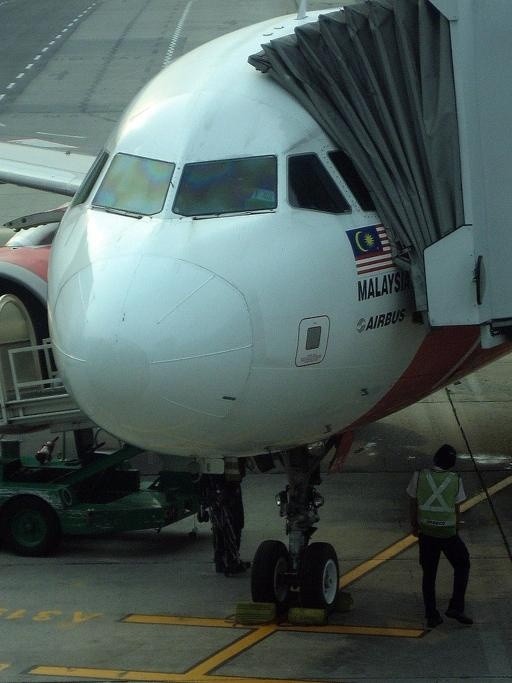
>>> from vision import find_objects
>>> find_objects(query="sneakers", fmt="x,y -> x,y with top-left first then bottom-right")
445,608 -> 474,627
216,560 -> 251,577
425,617 -> 446,628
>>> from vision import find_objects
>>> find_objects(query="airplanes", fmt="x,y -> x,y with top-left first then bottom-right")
0,0 -> 512,614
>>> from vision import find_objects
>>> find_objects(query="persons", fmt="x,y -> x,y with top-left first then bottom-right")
407,444 -> 472,628
211,474 -> 252,572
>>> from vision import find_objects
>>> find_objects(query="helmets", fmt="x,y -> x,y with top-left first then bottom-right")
434,442 -> 458,470
